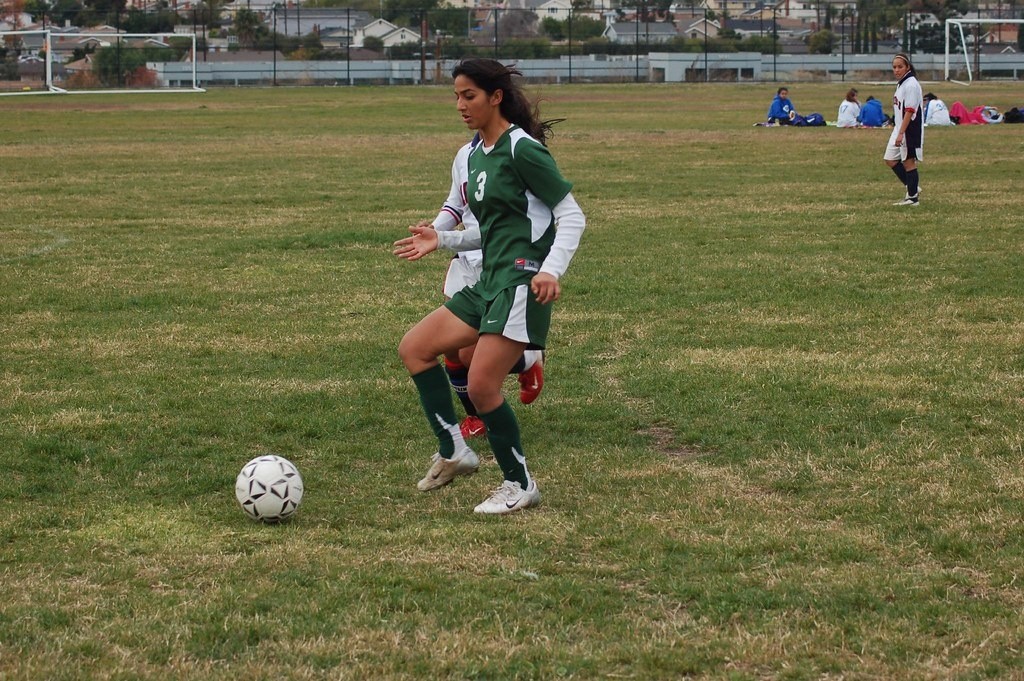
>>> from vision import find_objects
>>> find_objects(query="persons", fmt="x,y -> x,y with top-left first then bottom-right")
835,88 -> 889,127
922,93 -> 1005,127
393,57 -> 588,514
766,87 -> 796,126
882,54 -> 925,207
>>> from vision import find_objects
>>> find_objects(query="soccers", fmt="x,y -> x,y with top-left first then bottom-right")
232,454 -> 305,524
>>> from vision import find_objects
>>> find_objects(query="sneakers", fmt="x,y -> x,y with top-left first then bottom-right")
905,187 -> 922,198
475,480 -> 539,514
519,350 -> 545,405
893,197 -> 920,206
417,447 -> 480,490
459,415 -> 487,438
